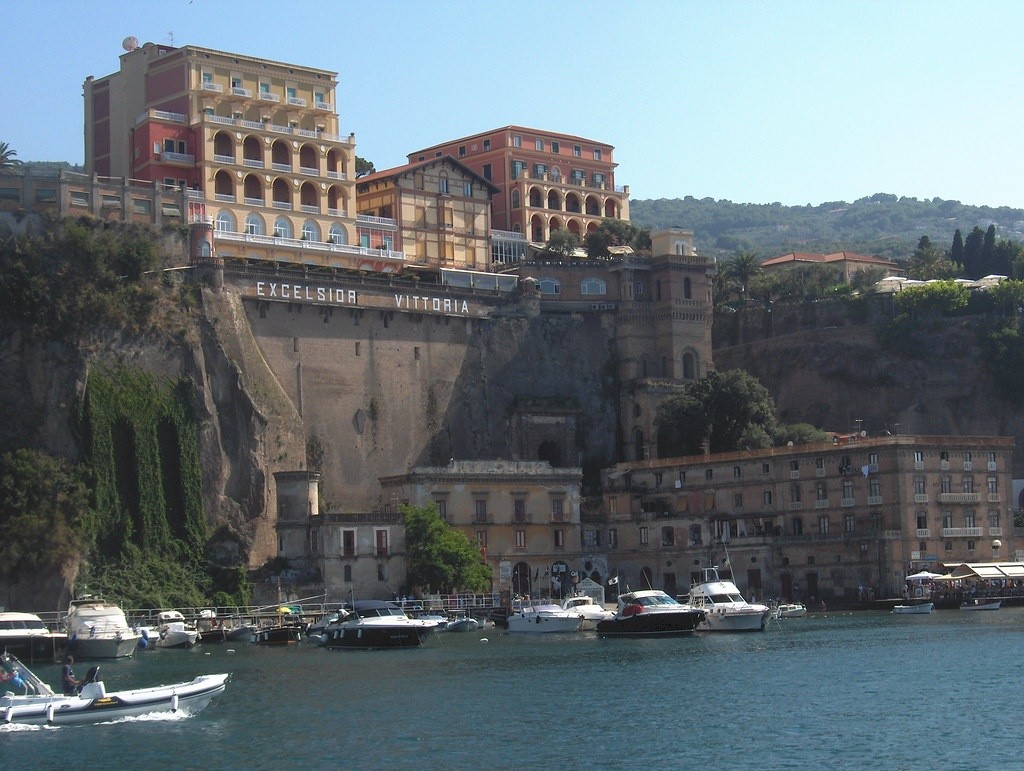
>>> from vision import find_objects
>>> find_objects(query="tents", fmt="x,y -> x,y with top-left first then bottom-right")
905,571 -> 943,583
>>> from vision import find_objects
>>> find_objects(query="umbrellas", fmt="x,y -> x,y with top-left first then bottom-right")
277,607 -> 291,613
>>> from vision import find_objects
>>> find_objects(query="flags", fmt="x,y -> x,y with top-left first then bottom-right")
609,575 -> 619,585
720,548 -> 731,567
534,566 -> 560,583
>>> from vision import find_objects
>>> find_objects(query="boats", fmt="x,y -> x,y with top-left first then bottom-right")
682,567 -> 770,631
63,598 -> 141,657
156,611 -> 199,647
309,600 -> 441,647
199,626 -> 229,642
149,608 -> 291,624
0,653 -> 228,728
443,609 -> 479,632
959,602 -> 1002,610
250,618 -> 302,644
773,602 -> 807,618
506,598 -> 585,632
563,595 -> 615,633
893,601 -> 933,614
595,589 -> 706,635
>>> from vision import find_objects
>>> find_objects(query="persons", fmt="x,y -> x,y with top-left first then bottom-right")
61,656 -> 84,694
394,593 -> 408,610
751,579 -> 1024,612
